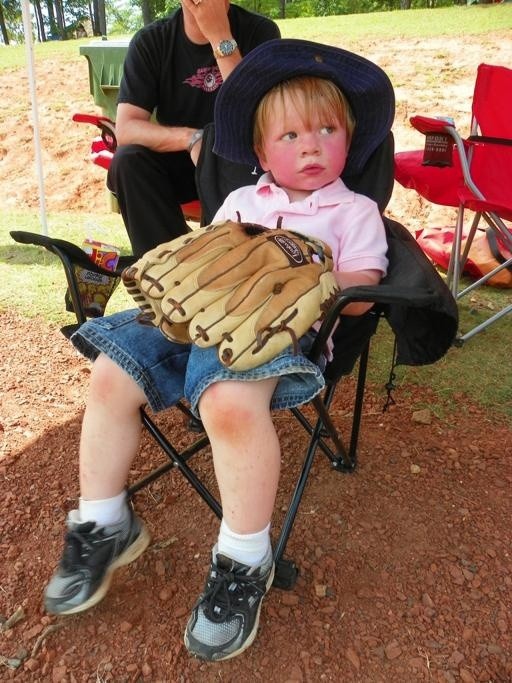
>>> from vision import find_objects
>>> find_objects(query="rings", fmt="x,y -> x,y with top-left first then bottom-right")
195,0 -> 202,6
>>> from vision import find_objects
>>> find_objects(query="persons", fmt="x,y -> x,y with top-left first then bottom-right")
106,0 -> 281,259
40,76 -> 391,663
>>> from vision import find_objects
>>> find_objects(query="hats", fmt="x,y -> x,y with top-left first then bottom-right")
212,40 -> 395,177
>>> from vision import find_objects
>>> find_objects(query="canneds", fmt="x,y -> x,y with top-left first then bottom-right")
423,116 -> 454,165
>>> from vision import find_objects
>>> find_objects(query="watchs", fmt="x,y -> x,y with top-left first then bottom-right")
187,128 -> 205,152
213,39 -> 237,59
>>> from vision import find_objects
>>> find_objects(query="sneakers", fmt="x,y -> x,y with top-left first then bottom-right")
184,542 -> 276,662
44,491 -> 153,615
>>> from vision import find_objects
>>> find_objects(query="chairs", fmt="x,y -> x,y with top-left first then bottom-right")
9,38 -> 458,591
72,113 -> 202,222
393,64 -> 512,349
